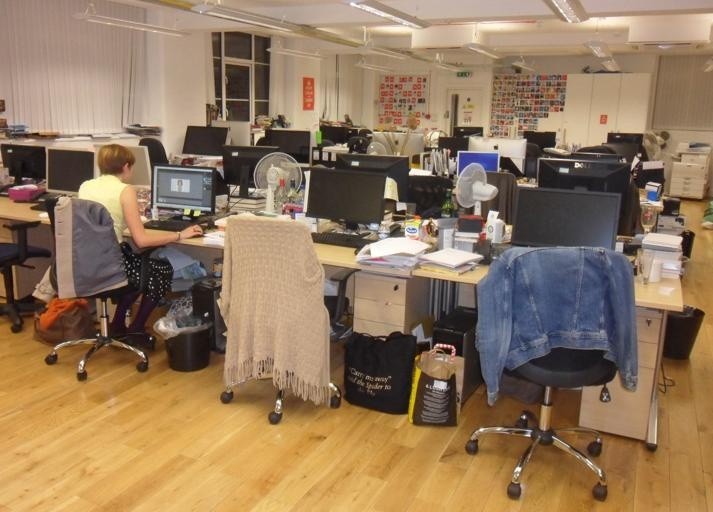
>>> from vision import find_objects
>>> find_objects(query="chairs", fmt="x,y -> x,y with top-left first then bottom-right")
317,139 -> 335,147
465,247 -> 637,501
43,196 -> 156,380
347,136 -> 368,153
220,214 -> 341,425
0,220 -> 51,333
358,129 -> 372,137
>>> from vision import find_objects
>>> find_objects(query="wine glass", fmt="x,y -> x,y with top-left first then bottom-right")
640,204 -> 658,234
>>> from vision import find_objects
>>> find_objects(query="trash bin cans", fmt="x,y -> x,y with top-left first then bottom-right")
160,316 -> 210,372
663,304 -> 705,360
681,229 -> 695,258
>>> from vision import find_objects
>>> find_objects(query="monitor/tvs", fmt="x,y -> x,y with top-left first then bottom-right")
349,129 -> 358,138
46,147 -> 97,197
510,187 -> 622,251
305,167 -> 386,238
372,132 -> 424,163
265,130 -> 312,168
536,157 -> 631,235
337,153 -> 409,234
457,150 -> 500,179
601,143 -> 639,165
0,144 -> 47,186
523,130 -> 556,150
181,125 -> 232,160
607,133 -> 643,152
468,135 -> 527,177
151,163 -> 216,224
453,127 -> 483,138
439,137 -> 468,159
319,126 -> 349,146
222,144 -> 280,199
93,144 -> 152,190
570,152 -> 623,162
211,119 -> 250,147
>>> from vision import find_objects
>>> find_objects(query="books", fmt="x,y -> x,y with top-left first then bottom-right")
417,247 -> 484,275
0,120 -> 30,138
126,123 -> 161,137
355,237 -> 432,268
641,232 -> 685,280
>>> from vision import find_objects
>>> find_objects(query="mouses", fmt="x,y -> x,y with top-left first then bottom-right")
193,230 -> 201,238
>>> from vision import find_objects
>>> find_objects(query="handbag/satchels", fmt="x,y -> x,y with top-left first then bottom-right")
408,344 -> 465,427
344,331 -> 417,414
32,308 -> 97,346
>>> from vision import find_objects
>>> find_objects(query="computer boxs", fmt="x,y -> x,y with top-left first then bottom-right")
192,276 -> 227,351
431,306 -> 484,414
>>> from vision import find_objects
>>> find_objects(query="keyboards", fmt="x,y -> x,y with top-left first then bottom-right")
143,220 -> 192,231
30,201 -> 48,211
311,232 -> 361,247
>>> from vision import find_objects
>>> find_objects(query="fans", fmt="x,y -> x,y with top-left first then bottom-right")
254,152 -> 302,216
456,162 -> 499,217
366,142 -> 387,155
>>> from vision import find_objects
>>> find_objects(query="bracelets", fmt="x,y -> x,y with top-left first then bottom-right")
176,232 -> 180,242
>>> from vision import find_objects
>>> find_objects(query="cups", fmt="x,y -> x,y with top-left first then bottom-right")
650,262 -> 664,282
636,248 -> 654,284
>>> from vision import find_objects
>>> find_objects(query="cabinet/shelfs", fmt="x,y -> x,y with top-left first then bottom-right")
622,72 -> 655,136
587,73 -> 621,146
668,153 -> 708,203
562,73 -> 594,152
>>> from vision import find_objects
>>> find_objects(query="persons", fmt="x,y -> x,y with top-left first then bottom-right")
344,114 -> 353,126
79,144 -> 203,351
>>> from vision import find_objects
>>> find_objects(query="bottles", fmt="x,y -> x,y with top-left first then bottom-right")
151,204 -> 159,221
378,221 -> 391,241
137,188 -> 145,216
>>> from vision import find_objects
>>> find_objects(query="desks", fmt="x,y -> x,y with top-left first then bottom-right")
225,202 -> 412,340
639,188 -> 665,234
0,184 -> 56,305
43,197 -> 238,353
412,211 -> 685,452
